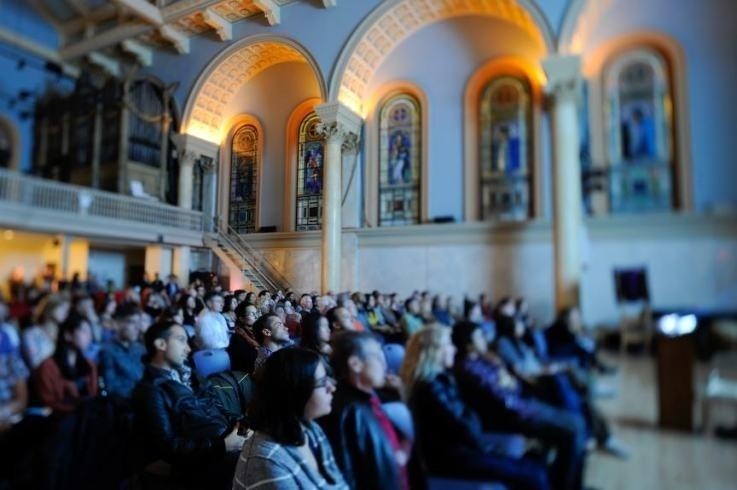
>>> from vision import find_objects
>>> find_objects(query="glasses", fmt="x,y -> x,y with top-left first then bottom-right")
314,378 -> 327,388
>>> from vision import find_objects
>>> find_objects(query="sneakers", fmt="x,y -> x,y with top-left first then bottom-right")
600,439 -> 631,457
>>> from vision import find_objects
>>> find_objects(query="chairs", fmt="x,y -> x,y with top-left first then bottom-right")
621,307 -> 652,354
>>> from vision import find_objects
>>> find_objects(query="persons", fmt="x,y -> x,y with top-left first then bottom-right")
390,133 -> 409,184
237,159 -> 252,201
1,266 -> 632,490
630,108 -> 650,195
305,147 -> 322,192
492,125 -> 519,221
